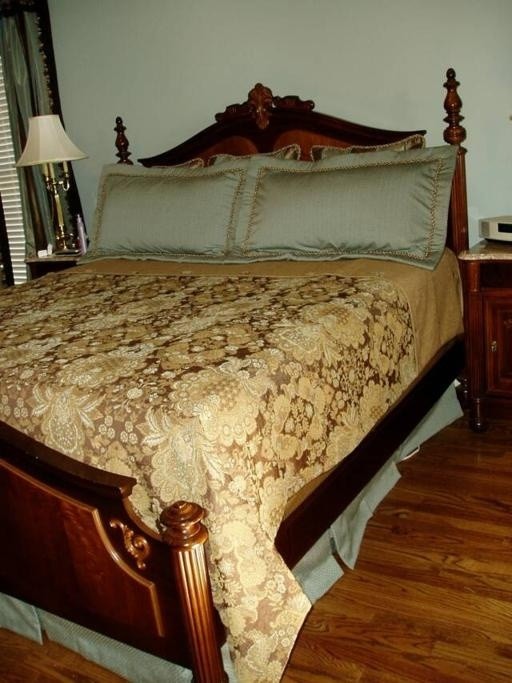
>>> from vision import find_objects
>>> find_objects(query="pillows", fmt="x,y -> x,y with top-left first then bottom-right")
235,142 -> 460,270
84,162 -> 252,267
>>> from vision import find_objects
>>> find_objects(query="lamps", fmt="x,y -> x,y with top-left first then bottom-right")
12,114 -> 90,256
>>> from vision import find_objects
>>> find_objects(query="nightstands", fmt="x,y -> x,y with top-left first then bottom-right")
0,67 -> 467,683
458,239 -> 512,431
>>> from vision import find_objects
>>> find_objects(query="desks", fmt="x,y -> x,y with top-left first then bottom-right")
24,255 -> 78,281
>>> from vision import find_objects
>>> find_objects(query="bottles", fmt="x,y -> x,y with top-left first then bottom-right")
76,214 -> 89,257
